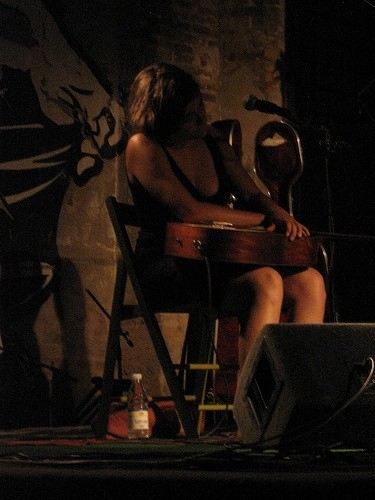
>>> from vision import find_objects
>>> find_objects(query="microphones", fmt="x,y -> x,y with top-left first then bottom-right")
243,94 -> 302,124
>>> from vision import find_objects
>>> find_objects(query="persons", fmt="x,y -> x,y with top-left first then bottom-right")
126,63 -> 327,385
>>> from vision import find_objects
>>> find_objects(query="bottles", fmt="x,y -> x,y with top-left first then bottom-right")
128,373 -> 150,441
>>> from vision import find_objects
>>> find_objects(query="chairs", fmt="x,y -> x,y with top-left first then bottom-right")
105,195 -> 200,441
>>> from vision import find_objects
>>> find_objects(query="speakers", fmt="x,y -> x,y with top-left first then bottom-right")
233,322 -> 375,453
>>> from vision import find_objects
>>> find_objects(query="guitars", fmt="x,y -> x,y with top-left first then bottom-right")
162,220 -> 375,270
